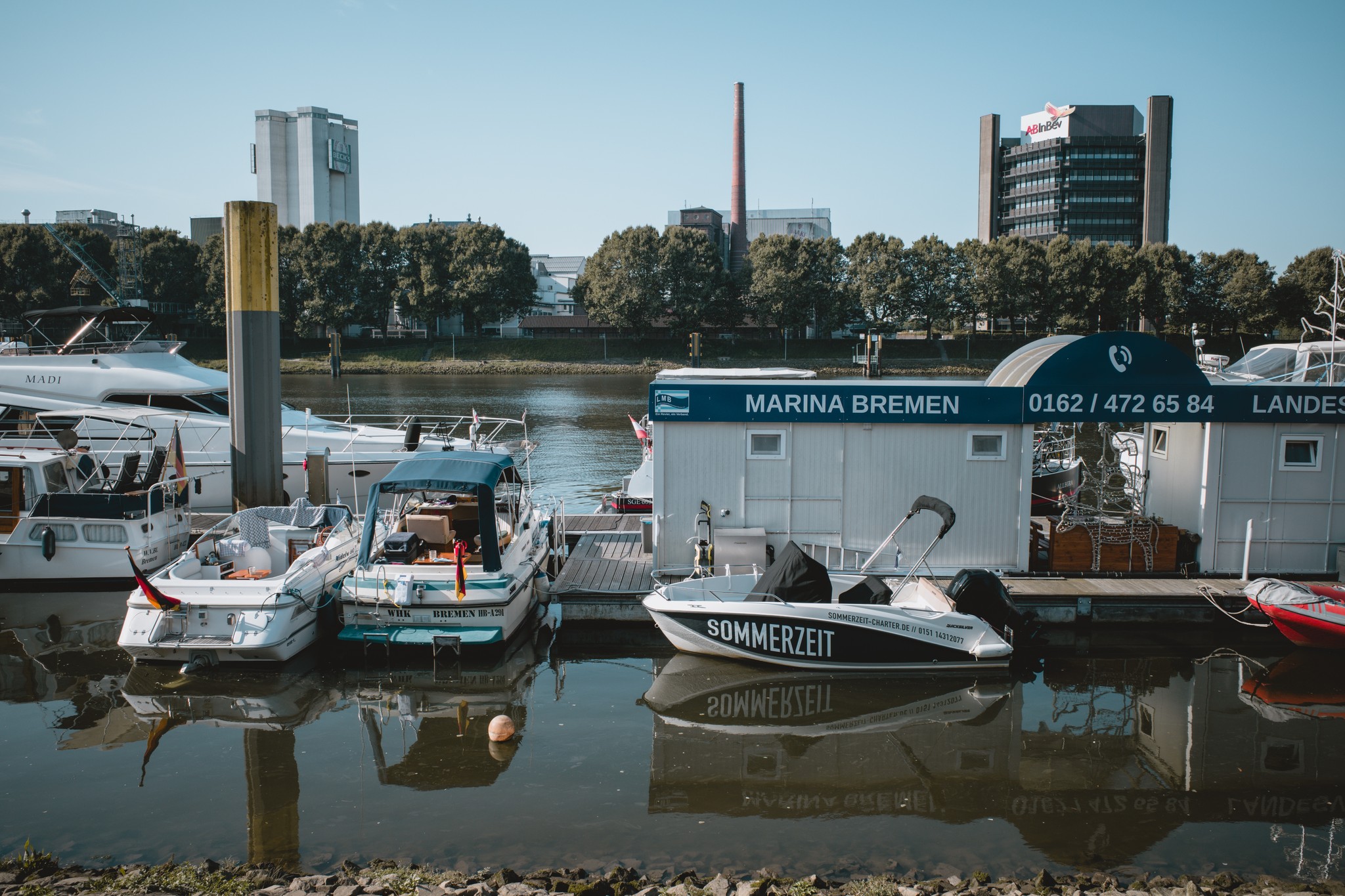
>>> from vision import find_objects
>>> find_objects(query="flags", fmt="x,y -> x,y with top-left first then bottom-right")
169,423 -> 188,496
628,415 -> 652,453
128,555 -> 181,611
455,546 -> 468,602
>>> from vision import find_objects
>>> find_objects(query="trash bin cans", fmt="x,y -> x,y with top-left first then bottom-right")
639,516 -> 653,553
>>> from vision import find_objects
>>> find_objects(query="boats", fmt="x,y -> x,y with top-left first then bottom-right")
1237,648 -> 1345,724
115,384 -> 400,664
0,305 -> 540,516
0,406 -> 224,587
1,583 -> 140,675
610,413 -> 653,514
1110,321 -> 1345,511
337,423 -> 552,658
119,638 -> 339,788
1031,420 -> 1084,517
640,494 -> 1050,673
335,603 -> 557,813
1243,577 -> 1345,650
635,652 -> 1047,737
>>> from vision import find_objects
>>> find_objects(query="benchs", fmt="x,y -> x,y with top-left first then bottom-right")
405,514 -> 457,554
168,557 -> 202,580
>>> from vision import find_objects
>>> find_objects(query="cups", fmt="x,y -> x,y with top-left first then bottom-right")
425,549 -> 430,557
429,550 -> 437,559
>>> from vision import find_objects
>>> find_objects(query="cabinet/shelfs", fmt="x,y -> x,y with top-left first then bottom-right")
195,536 -> 234,580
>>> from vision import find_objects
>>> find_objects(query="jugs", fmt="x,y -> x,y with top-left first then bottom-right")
455,539 -> 467,557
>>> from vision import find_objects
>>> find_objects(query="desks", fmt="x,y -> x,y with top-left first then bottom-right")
227,569 -> 271,580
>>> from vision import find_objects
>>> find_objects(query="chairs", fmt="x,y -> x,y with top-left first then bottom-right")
475,511 -> 516,553
86,450 -> 148,494
130,445 -> 168,492
